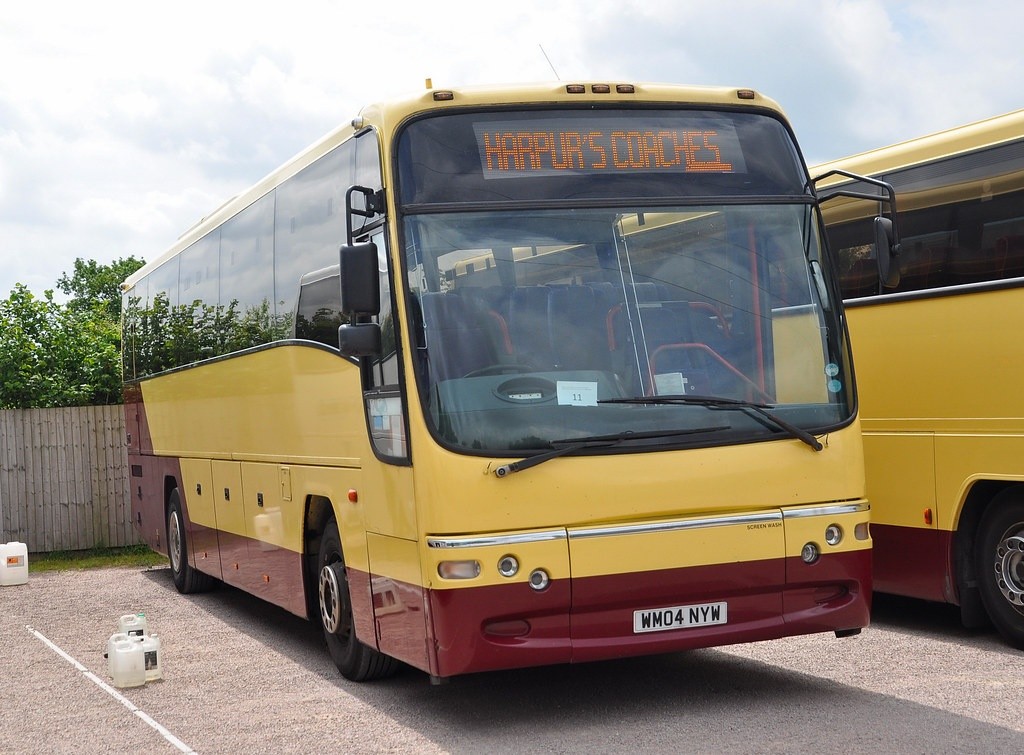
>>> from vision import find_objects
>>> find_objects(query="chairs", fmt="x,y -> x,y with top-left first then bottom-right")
419,284 -> 754,402
846,253 -> 982,292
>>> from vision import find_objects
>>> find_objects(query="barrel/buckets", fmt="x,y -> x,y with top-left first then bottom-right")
0,542 -> 29,586
107,632 -> 145,688
118,613 -> 148,635
137,634 -> 161,682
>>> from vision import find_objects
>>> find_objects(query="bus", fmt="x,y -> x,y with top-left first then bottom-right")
116,77 -> 1023,685
